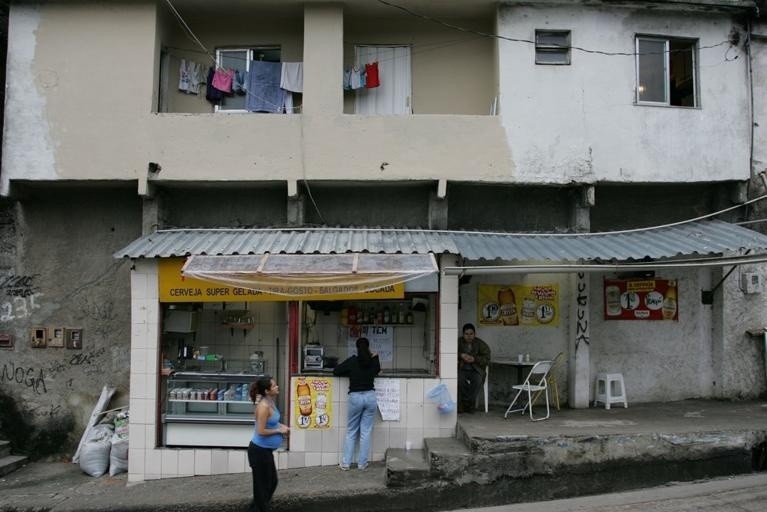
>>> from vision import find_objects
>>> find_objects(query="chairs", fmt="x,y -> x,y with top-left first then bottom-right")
504,359 -> 554,421
474,364 -> 489,413
523,351 -> 564,412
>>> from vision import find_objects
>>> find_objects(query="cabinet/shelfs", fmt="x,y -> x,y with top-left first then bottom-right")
344,299 -> 416,327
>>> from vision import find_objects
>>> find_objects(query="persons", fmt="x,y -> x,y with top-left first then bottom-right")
459,323 -> 491,414
333,337 -> 380,470
249,377 -> 290,511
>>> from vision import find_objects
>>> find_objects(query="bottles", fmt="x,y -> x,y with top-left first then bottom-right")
497,285 -> 519,326
661,278 -> 677,320
234,384 -> 250,402
341,301 -> 414,325
297,378 -> 312,416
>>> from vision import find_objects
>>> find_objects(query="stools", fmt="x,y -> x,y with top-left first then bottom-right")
593,372 -> 628,410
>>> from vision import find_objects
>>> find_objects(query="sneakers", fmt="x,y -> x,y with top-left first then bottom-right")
340,463 -> 350,471
358,463 -> 368,470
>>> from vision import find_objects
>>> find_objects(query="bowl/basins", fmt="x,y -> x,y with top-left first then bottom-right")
168,304 -> 178,310
